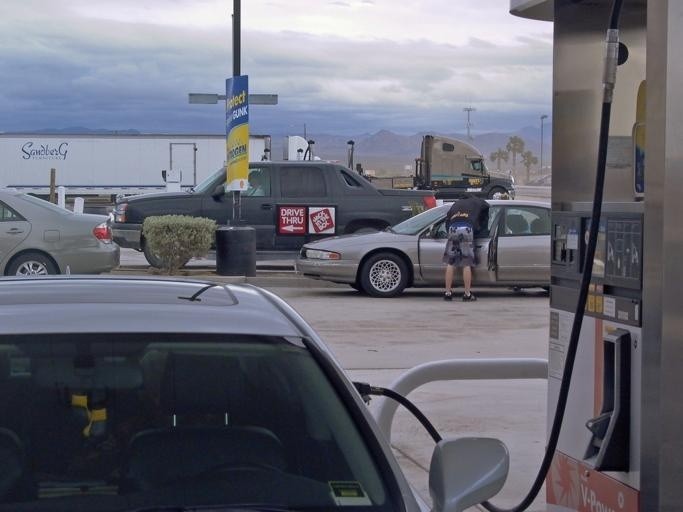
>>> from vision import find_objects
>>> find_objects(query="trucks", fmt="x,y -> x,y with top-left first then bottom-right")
0,133 -> 320,198
365,135 -> 515,201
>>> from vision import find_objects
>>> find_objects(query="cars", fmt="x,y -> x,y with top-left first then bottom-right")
295,200 -> 551,298
108,160 -> 436,268
0,277 -> 510,512
0,188 -> 119,276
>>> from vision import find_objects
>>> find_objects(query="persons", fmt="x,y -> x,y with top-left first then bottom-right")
442,194 -> 489,302
246,171 -> 267,196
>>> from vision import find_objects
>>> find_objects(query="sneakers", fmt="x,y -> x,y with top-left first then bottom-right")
463,291 -> 477,302
444,290 -> 452,301
